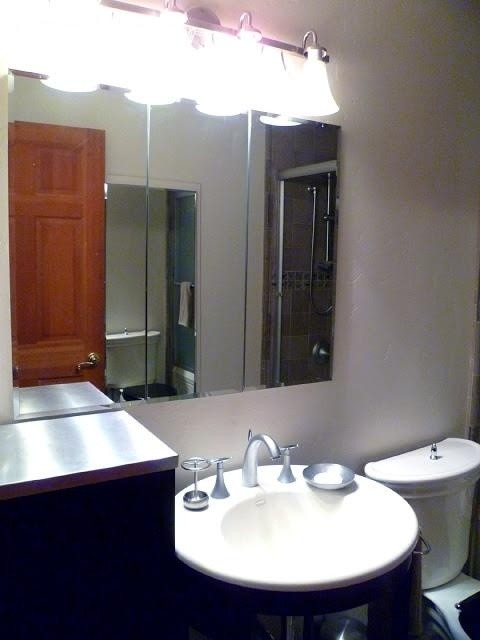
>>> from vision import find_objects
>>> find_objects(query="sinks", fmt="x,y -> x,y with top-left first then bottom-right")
174,464 -> 419,616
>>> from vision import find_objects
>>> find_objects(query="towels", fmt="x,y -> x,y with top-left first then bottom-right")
177,281 -> 193,329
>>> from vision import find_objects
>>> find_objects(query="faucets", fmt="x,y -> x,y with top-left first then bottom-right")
241,434 -> 281,485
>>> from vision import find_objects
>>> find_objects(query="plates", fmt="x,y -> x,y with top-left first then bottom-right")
302,462 -> 356,491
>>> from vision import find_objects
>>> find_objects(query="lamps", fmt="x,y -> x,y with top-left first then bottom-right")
42,6 -> 341,124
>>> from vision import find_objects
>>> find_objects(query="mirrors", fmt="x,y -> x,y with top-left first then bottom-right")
7,70 -> 344,423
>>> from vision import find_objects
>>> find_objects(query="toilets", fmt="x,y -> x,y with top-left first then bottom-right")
105,330 -> 179,401
363,435 -> 479,640
